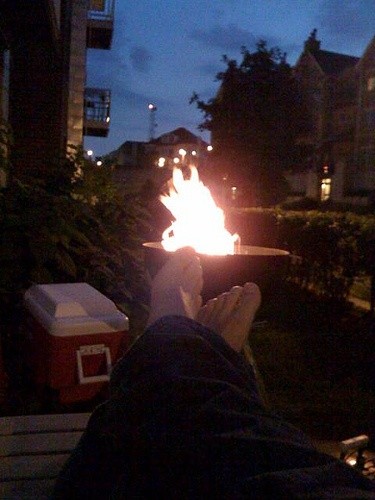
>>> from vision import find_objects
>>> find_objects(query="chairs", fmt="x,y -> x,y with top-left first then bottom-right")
1,406 -> 92,499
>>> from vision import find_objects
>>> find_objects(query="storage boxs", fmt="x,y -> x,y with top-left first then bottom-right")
21,283 -> 129,402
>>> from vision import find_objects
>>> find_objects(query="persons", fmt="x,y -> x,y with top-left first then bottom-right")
49,246 -> 375,500
